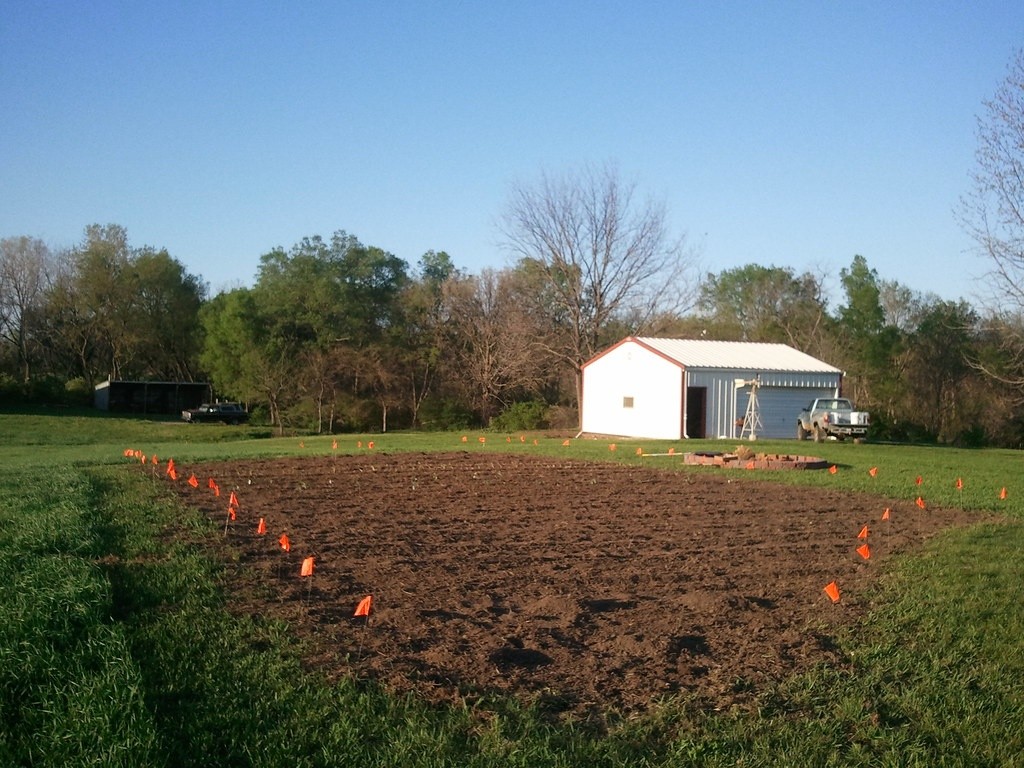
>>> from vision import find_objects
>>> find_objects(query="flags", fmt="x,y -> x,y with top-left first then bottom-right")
916,476 -> 922,485
333,439 -> 374,449
564,440 -> 754,469
461,434 -> 539,446
210,477 -> 220,495
354,597 -> 370,616
300,557 -> 314,576
188,475 -> 199,487
855,544 -> 870,560
824,582 -> 840,601
869,466 -> 878,477
858,526 -> 867,537
126,449 -> 157,465
917,496 -> 925,509
830,466 -> 837,474
957,479 -> 963,489
230,493 -> 238,520
279,535 -> 290,552
1000,488 -> 1008,499
257,519 -> 266,535
883,510 -> 889,519
167,459 -> 177,479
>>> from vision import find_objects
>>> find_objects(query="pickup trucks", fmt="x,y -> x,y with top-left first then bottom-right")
181,402 -> 249,424
798,398 -> 870,444
